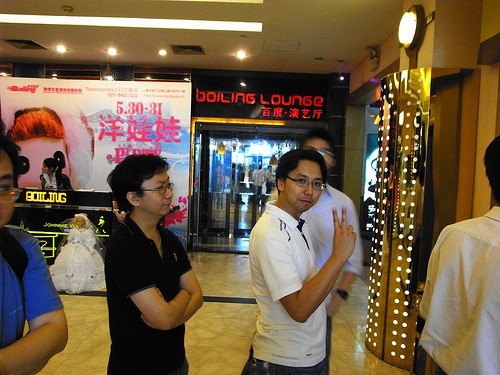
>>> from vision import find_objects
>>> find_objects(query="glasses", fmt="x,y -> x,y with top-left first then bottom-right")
0,186 -> 22,203
311,146 -> 333,157
135,180 -> 174,196
284,173 -> 327,192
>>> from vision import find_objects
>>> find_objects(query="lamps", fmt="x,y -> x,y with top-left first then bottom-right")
397,5 -> 426,55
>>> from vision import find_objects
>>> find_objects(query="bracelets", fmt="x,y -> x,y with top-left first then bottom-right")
113,208 -> 119,213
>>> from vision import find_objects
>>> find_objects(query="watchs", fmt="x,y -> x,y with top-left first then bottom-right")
336,288 -> 349,300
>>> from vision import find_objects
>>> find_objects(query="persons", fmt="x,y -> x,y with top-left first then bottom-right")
249,148 -> 357,375
419,136 -> 500,375
40,158 -> 72,209
112,193 -> 128,236
0,134 -> 68,375
104,152 -> 204,375
229,163 -> 273,207
241,128 -> 364,375
48,212 -> 107,294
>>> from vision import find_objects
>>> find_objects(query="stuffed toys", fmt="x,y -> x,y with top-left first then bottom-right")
0,101 -> 95,191
